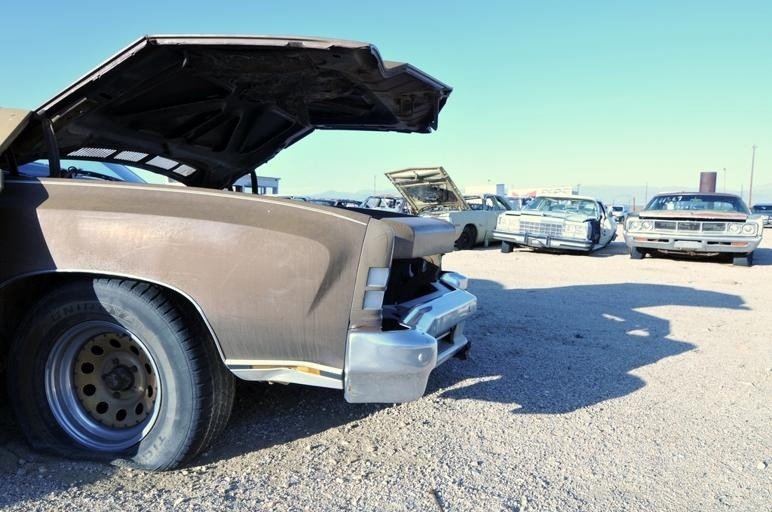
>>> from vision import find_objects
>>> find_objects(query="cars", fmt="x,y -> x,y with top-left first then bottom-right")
622,191 -> 764,268
0,33 -> 481,472
383,163 -> 511,250
491,194 -> 617,253
